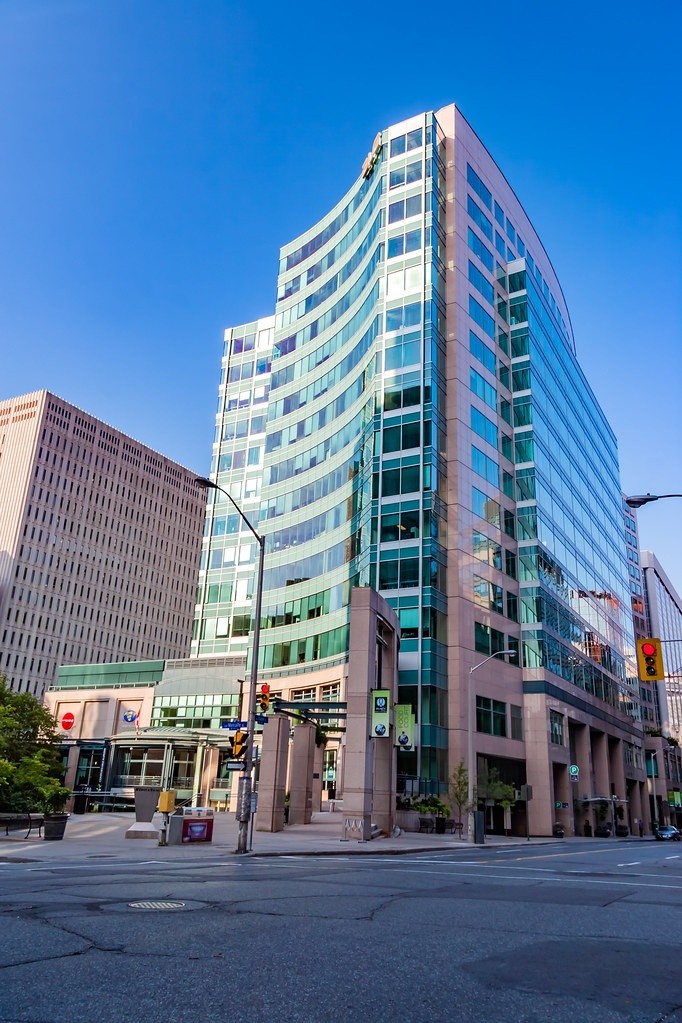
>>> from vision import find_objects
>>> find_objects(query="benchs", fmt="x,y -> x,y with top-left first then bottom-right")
0,812 -> 44,839
445,820 -> 463,834
418,817 -> 437,834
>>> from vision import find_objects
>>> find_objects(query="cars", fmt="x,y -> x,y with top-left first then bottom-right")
655,826 -> 682,841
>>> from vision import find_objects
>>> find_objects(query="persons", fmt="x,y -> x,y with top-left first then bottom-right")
584,820 -> 591,837
639,820 -> 643,837
553,822 -> 564,836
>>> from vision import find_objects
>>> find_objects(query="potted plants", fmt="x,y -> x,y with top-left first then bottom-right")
34,786 -> 72,841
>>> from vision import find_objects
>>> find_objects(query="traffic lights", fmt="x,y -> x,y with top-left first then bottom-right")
227,736 -> 238,759
261,685 -> 271,711
235,732 -> 250,758
634,638 -> 665,682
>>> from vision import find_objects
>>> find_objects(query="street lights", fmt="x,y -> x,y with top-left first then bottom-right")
192,475 -> 266,856
651,745 -> 676,828
466,649 -> 518,843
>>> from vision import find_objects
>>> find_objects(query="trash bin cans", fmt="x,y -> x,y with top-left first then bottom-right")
165,815 -> 184,845
183,807 -> 216,842
329,802 -> 335,812
